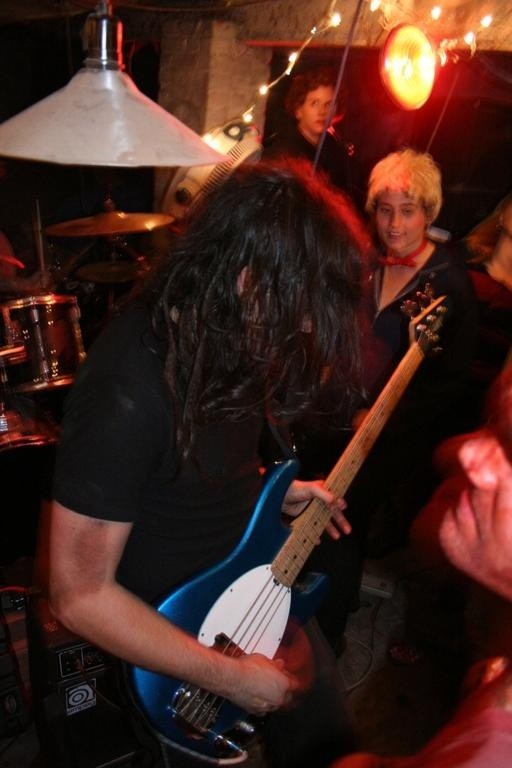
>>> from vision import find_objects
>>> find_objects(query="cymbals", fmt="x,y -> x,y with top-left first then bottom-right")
77,260 -> 153,281
42,211 -> 175,236
0,348 -> 64,448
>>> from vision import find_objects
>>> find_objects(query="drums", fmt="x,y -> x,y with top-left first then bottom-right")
1,295 -> 86,391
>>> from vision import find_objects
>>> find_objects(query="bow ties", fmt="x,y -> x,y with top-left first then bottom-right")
376,238 -> 430,268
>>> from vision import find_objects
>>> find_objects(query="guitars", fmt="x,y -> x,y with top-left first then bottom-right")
133,284 -> 446,760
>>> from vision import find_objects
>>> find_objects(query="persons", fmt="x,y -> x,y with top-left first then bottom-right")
318,149 -> 510,766
38,162 -> 367,765
265,68 -> 352,183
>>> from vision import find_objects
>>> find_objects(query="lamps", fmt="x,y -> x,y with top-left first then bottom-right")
0,0 -> 231,167
379,24 -> 479,111
